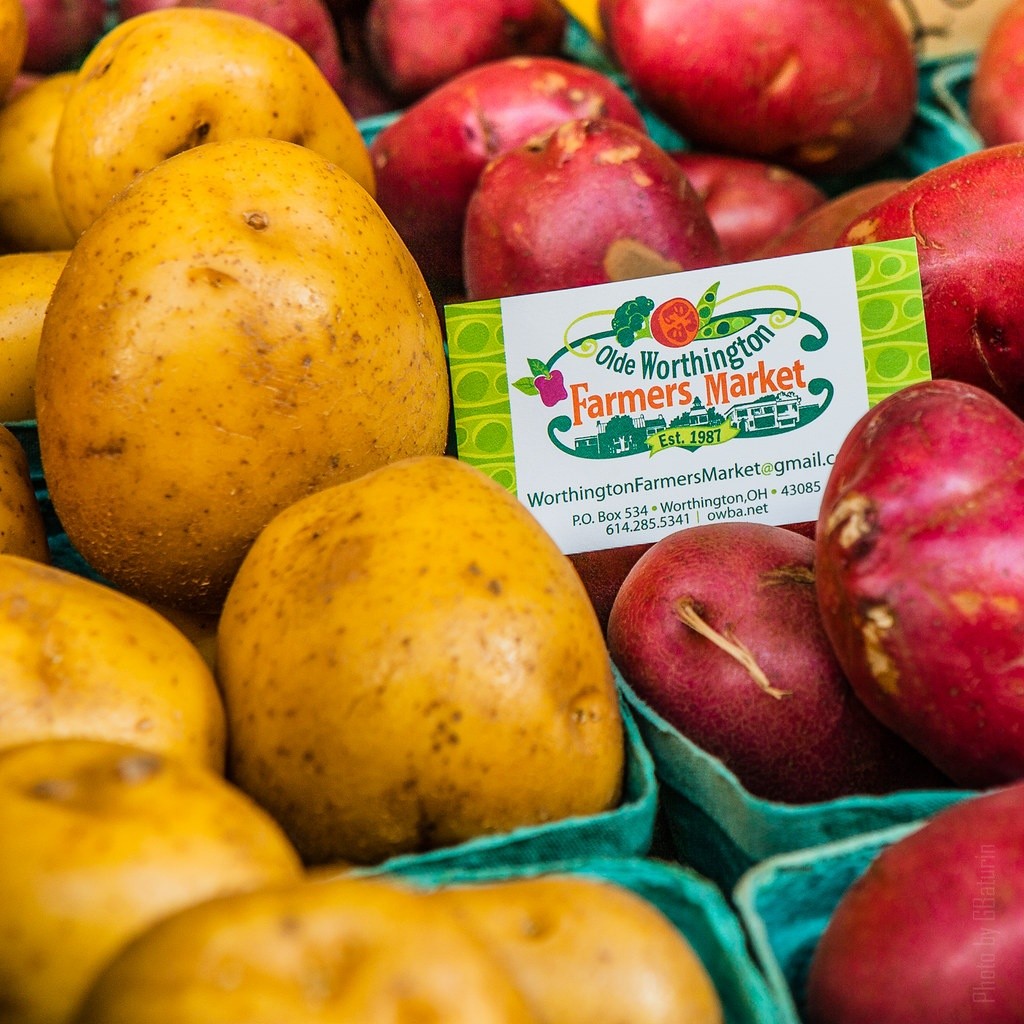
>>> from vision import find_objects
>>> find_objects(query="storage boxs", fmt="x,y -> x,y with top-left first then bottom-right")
331,18 -> 1024,1024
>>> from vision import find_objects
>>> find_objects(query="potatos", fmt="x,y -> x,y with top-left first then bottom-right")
2,0 -> 1024,1024
